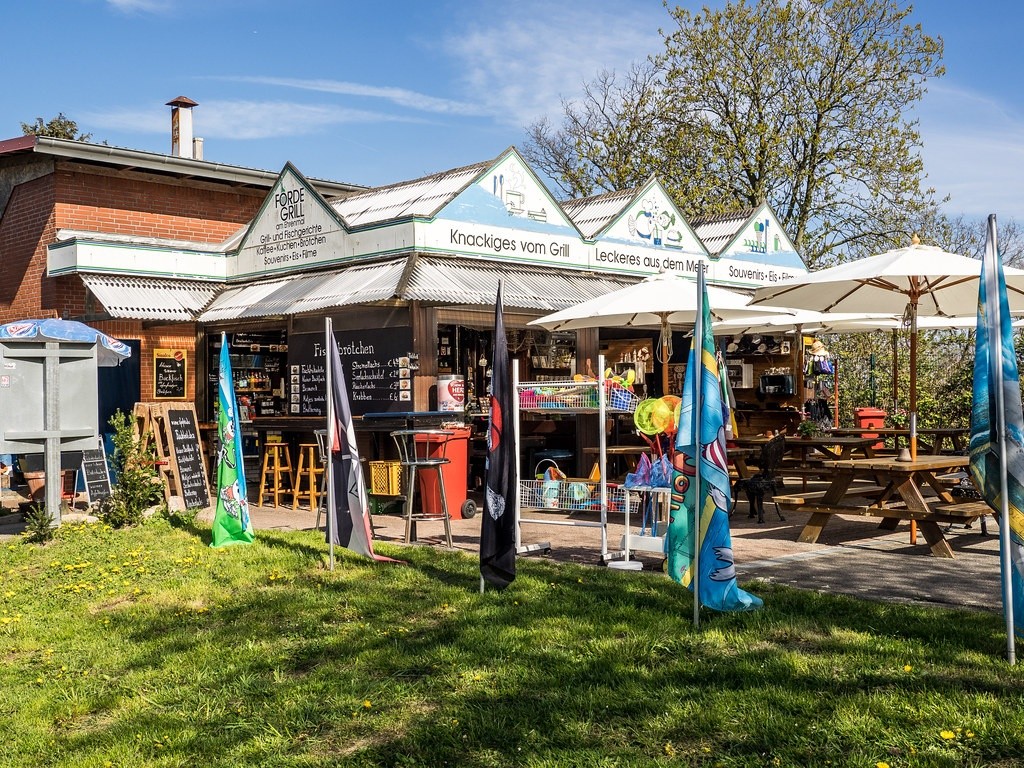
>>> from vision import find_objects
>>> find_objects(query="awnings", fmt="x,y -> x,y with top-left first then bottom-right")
77,256 -> 407,323
406,255 -> 662,311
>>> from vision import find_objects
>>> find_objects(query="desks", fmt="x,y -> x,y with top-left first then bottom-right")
727,435 -> 879,494
796,455 -> 1000,558
583,446 -> 765,515
825,428 -> 980,504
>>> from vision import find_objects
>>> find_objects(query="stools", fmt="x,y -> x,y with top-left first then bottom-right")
258,443 -> 301,509
292,444 -> 328,511
314,430 -> 328,532
389,429 -> 454,548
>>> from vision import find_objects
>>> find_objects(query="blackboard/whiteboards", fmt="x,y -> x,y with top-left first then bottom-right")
162,400 -> 211,511
153,349 -> 187,399
81,437 -> 113,505
288,326 -> 414,414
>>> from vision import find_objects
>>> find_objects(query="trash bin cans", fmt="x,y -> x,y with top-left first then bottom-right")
851,405 -> 887,448
413,425 -> 477,520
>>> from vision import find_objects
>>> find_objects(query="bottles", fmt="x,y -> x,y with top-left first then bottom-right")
232,371 -> 269,389
257,397 -> 275,416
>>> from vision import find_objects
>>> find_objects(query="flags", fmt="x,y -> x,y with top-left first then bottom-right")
965,213 -> 1023,639
211,334 -> 256,546
479,280 -> 520,591
664,266 -> 765,612
325,328 -> 408,569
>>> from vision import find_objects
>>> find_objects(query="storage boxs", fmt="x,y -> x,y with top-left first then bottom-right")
369,460 -> 406,495
367,489 -> 403,514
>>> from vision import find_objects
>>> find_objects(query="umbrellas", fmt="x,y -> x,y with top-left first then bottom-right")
1,318 -> 132,370
524,232 -> 1024,546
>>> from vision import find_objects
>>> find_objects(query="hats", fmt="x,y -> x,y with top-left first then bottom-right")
726,334 -> 785,354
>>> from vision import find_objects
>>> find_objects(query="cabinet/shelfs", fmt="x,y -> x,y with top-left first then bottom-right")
512,355 -> 642,567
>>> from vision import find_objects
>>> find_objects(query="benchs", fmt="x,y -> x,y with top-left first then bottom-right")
721,457 -> 998,559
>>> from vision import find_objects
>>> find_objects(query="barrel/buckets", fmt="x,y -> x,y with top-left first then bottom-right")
24,469 -> 74,498
